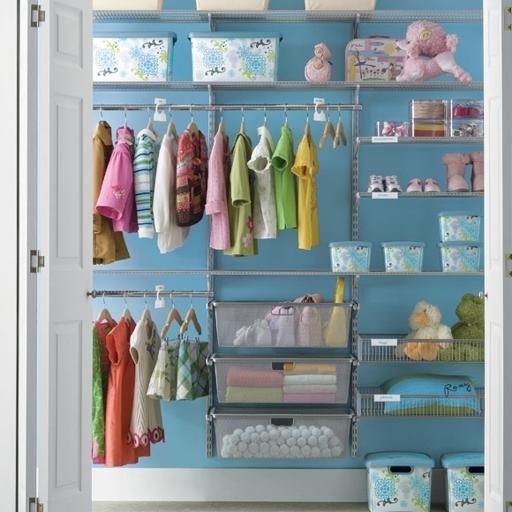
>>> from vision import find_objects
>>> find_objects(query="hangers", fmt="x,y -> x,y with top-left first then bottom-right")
90,287 -> 203,340
93,101 -> 349,154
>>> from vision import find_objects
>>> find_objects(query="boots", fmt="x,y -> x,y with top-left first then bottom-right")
468,152 -> 484,191
441,152 -> 470,192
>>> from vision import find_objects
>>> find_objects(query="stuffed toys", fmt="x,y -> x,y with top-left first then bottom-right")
393,302 -> 453,360
394,21 -> 474,84
439,293 -> 483,361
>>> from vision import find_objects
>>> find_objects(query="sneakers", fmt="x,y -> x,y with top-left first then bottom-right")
406,177 -> 423,192
367,175 -> 384,193
385,175 -> 403,192
424,178 -> 440,192
393,122 -> 409,137
381,121 -> 396,137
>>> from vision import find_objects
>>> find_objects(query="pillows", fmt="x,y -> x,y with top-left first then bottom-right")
380,373 -> 481,417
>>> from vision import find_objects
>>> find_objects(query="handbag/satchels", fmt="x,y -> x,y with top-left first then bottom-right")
323,275 -> 347,347
231,320 -> 272,347
295,295 -> 322,346
267,299 -> 296,347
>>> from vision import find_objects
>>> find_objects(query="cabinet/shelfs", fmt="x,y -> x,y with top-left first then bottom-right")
89,7 -> 483,459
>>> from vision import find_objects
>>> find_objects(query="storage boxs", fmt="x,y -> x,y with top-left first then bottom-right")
92,29 -> 283,84
363,450 -> 484,512
207,298 -> 355,456
